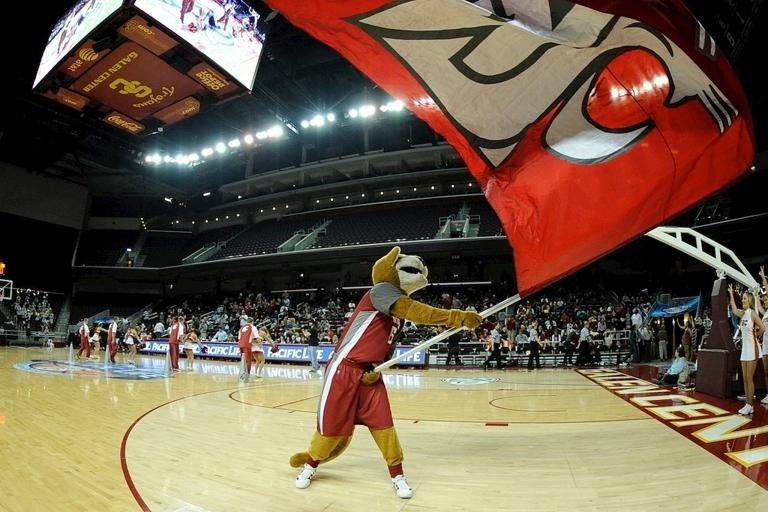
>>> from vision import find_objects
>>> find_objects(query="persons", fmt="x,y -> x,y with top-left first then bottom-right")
179,0 -> 265,41
0,260 -> 767,418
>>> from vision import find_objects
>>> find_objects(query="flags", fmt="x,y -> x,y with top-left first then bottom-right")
264,0 -> 757,301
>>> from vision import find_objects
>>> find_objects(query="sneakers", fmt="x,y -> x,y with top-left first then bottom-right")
391,474 -> 413,498
738,403 -> 754,414
761,394 -> 768,404
295,462 -> 316,488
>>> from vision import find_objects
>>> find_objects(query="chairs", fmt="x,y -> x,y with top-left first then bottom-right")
132,226 -> 193,267
195,196 -> 504,262
181,302 -> 623,346
1,288 -> 68,334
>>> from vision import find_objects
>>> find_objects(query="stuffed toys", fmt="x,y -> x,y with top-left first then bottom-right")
290,246 -> 483,499
